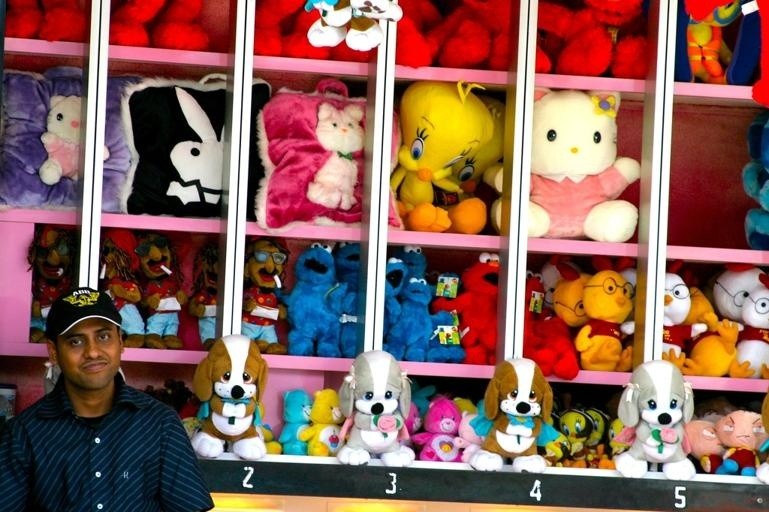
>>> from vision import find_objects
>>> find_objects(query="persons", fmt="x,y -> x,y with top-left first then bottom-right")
0,286 -> 215,512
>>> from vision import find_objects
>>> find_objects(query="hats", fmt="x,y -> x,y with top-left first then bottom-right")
46,287 -> 122,336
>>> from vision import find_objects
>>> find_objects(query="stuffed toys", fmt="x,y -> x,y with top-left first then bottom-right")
0,0 -> 769,487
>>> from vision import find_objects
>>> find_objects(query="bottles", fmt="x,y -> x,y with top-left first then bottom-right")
0,384 -> 19,422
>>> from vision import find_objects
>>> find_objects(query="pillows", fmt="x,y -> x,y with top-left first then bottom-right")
1,64 -> 141,214
121,70 -> 273,219
255,79 -> 404,235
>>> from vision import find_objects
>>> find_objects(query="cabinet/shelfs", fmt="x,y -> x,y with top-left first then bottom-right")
373,1 -> 530,380
654,0 -> 768,392
231,0 -> 388,373
0,0 -> 100,357
513,0 -> 669,389
1,344 -> 768,511
90,0 -> 244,372
